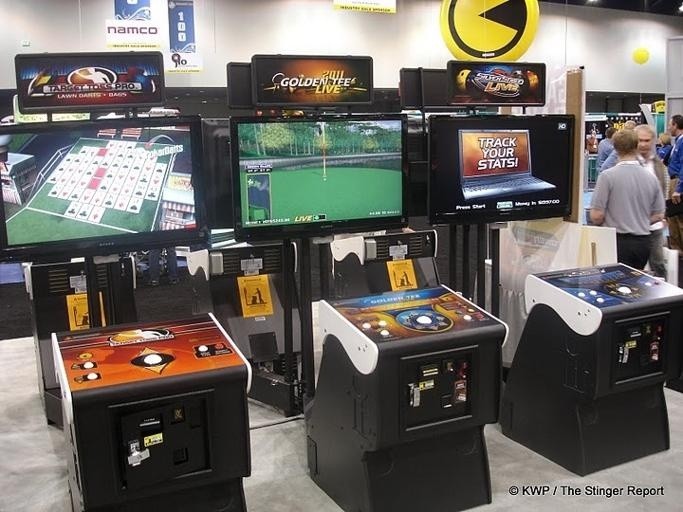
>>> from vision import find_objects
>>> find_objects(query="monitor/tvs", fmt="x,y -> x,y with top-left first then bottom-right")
428,114 -> 576,225
0,115 -> 209,262
230,114 -> 409,242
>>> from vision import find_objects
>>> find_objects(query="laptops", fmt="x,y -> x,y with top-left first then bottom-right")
458,129 -> 557,202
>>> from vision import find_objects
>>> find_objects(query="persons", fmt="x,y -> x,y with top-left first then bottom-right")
586,115 -> 683,281
